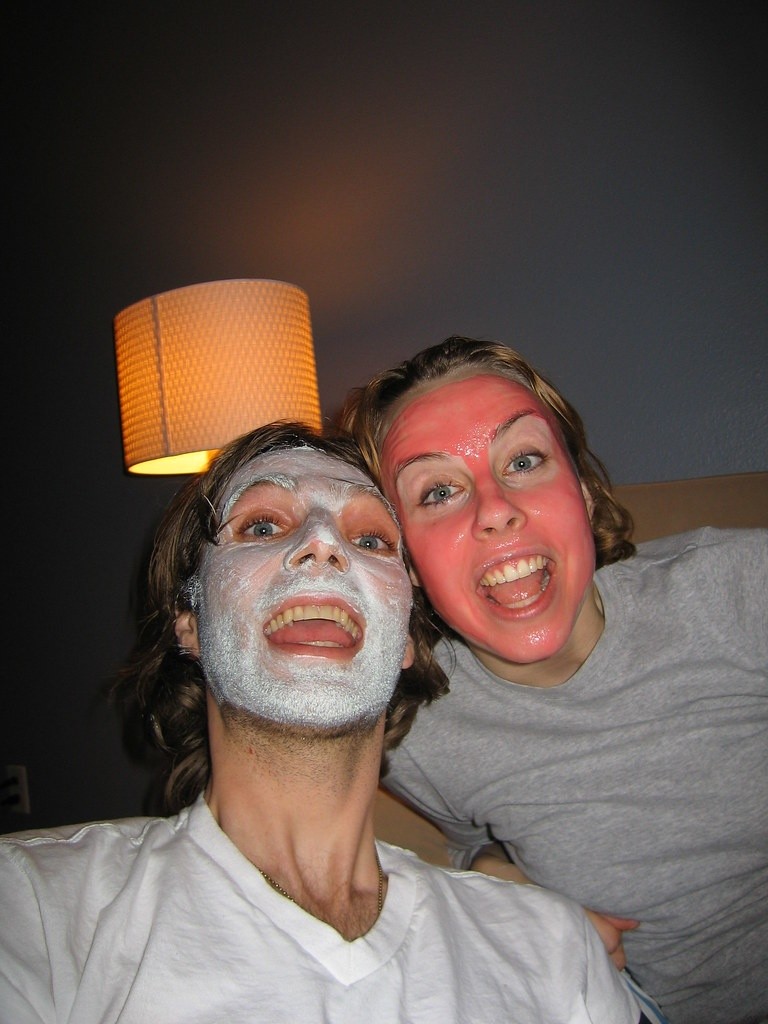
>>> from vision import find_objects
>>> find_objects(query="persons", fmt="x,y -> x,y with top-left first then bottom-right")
0,417 -> 641,1024
342,333 -> 768,1024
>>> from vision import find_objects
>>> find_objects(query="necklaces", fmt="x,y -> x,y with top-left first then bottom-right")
242,853 -> 383,942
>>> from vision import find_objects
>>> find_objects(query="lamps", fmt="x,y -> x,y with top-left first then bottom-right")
113,275 -> 323,477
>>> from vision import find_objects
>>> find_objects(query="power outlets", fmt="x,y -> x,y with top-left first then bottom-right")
5,764 -> 32,816
